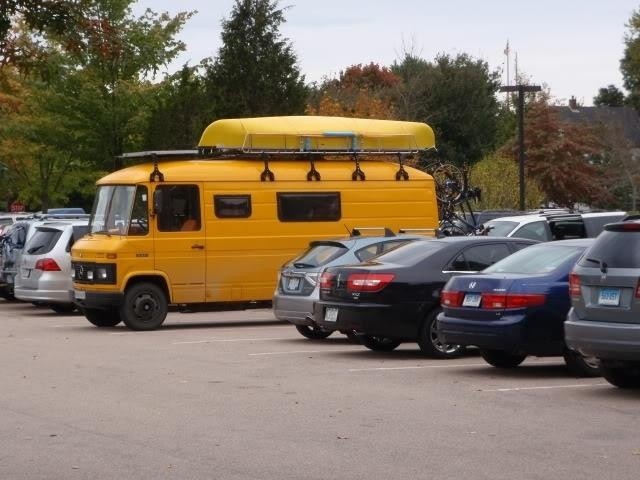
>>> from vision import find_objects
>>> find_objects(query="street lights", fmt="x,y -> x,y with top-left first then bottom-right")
498,85 -> 542,211
